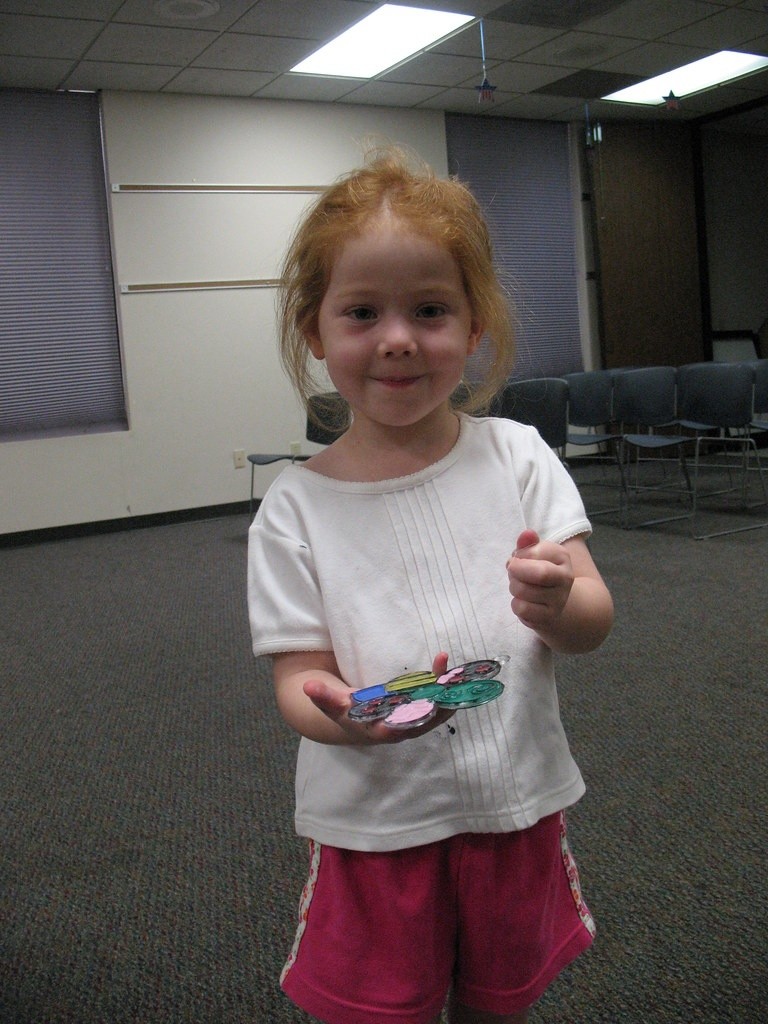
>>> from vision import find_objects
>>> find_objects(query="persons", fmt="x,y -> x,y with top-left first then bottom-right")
249,142 -> 618,1023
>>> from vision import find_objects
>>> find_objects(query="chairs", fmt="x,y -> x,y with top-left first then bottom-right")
448,359 -> 768,540
248,391 -> 351,523
